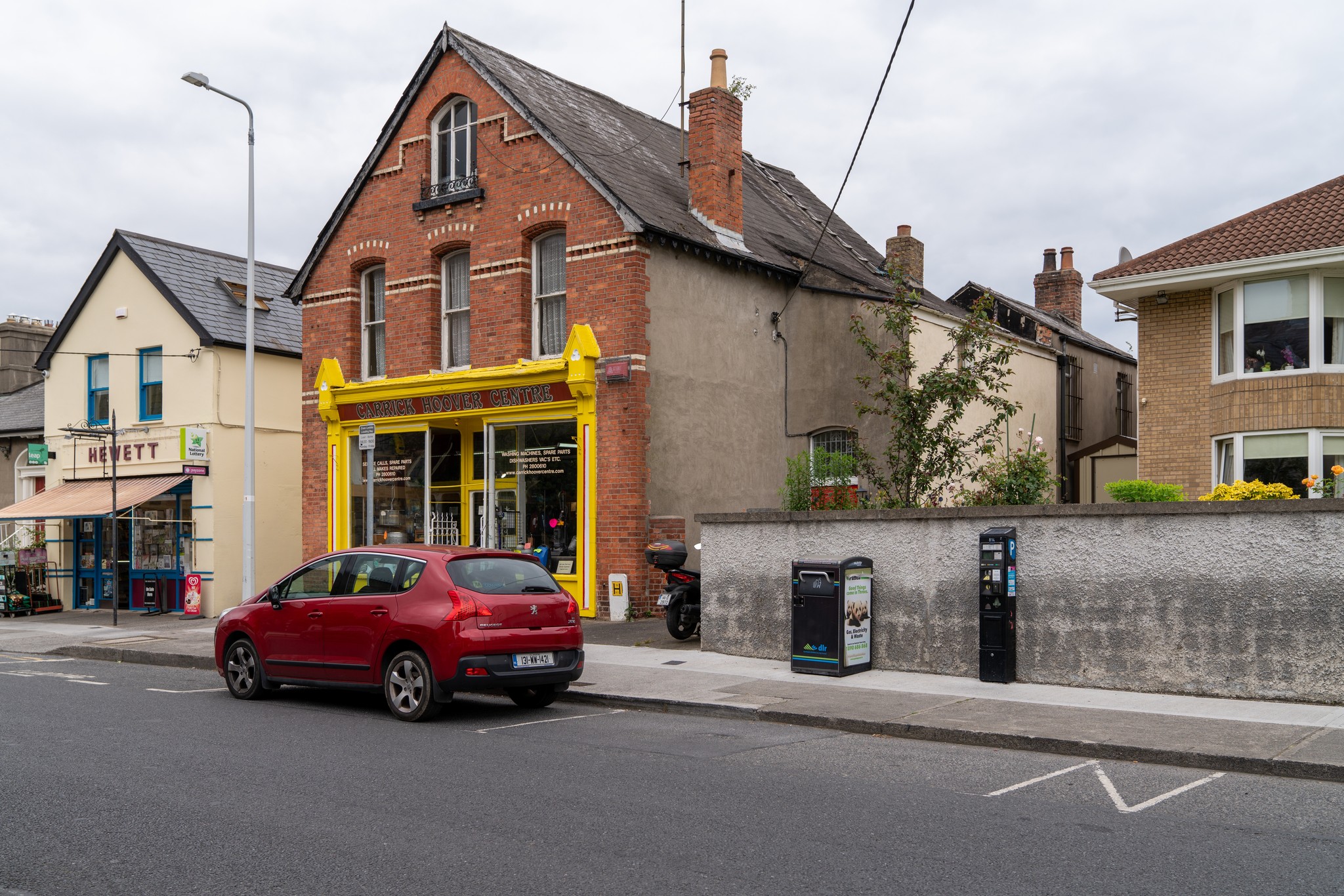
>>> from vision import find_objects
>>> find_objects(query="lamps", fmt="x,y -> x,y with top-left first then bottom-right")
1155,290 -> 1168,305
855,485 -> 868,504
116,426 -> 150,436
64,428 -> 124,439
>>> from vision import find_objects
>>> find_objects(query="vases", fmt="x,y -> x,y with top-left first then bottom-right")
1284,366 -> 1294,370
1262,367 -> 1270,372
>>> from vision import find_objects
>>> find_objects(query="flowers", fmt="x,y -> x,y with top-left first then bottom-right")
1280,348 -> 1293,369
1256,346 -> 1271,369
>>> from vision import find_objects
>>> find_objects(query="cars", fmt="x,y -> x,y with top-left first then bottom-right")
214,543 -> 585,722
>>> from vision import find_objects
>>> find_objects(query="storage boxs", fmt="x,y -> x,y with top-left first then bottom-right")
644,540 -> 688,566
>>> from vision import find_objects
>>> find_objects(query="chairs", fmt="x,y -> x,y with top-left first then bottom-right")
357,567 -> 393,594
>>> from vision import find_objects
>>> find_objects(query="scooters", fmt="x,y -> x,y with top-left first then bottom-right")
644,540 -> 701,640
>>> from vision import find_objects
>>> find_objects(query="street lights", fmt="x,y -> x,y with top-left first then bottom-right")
181,71 -> 256,601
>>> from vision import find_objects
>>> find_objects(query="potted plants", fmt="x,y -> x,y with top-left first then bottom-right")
1244,357 -> 1259,373
